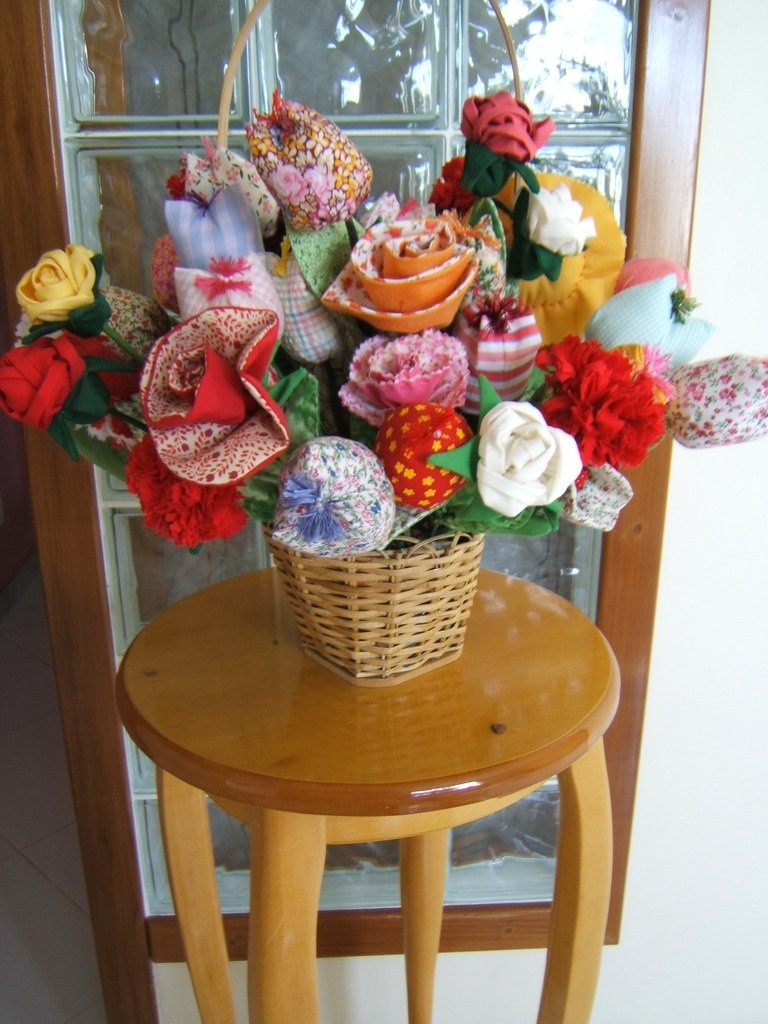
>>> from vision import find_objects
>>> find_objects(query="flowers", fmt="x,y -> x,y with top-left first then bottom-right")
0,92 -> 767,557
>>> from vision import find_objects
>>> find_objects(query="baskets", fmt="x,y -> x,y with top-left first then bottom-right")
217,0 -> 523,688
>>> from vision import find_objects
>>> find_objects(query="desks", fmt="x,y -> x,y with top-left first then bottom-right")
115,568 -> 622,1024
